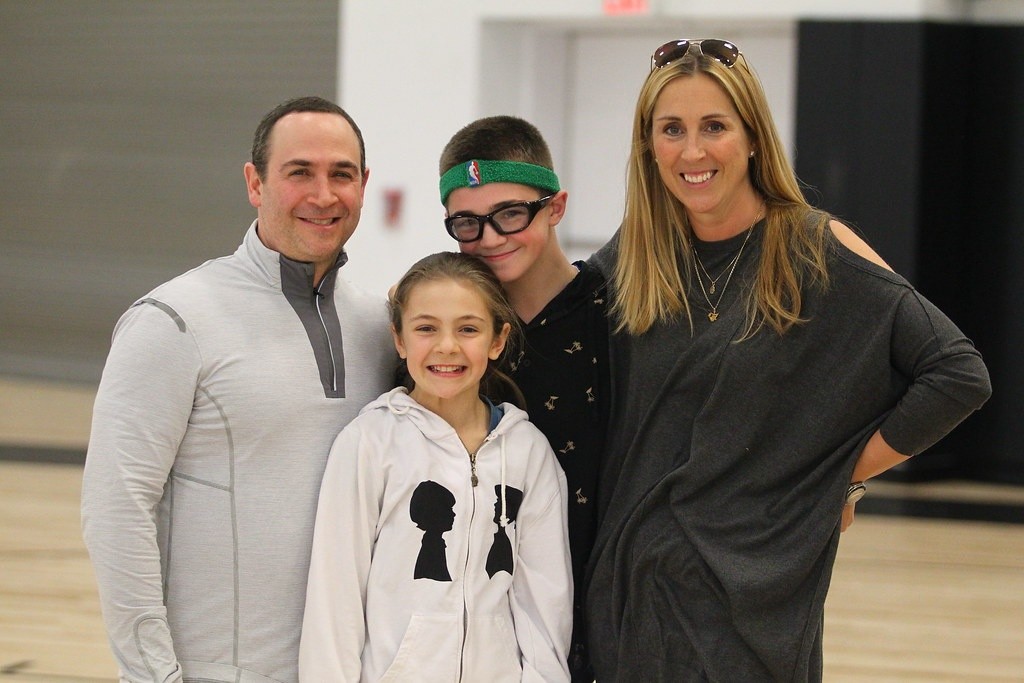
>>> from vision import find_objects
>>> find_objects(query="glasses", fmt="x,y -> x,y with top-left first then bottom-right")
651,38 -> 747,74
445,193 -> 557,243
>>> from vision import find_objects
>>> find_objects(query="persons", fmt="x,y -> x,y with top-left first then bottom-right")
298,252 -> 574,683
438,115 -> 610,683
389,38 -> 992,683
81,96 -> 398,683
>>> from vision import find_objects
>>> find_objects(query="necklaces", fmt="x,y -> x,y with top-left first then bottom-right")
693,201 -> 764,322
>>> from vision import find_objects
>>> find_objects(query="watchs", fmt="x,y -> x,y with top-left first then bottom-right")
846,482 -> 866,505
